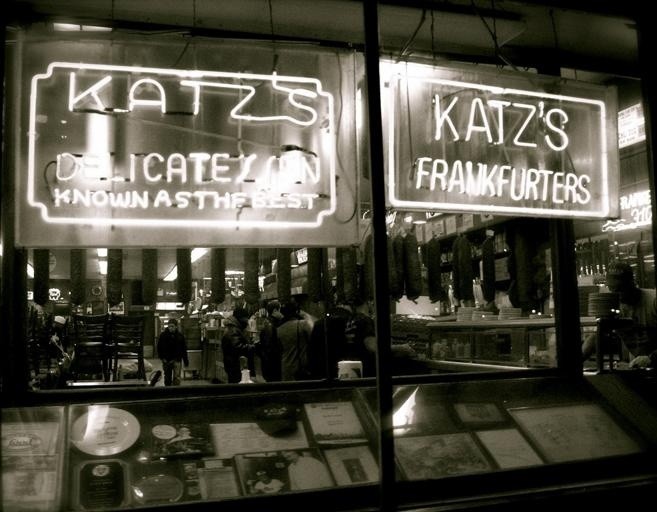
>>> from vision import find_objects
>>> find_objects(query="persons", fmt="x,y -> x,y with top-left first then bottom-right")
157,319 -> 189,387
245,469 -> 289,495
279,449 -> 334,492
579,263 -> 656,367
218,291 -> 415,383
159,425 -> 212,456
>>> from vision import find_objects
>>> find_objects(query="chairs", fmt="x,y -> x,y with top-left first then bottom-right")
69,312 -> 148,380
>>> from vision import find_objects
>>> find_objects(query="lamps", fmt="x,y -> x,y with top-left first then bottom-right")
259,249 -> 300,274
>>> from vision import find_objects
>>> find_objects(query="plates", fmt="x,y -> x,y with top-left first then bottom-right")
67,406 -> 143,458
455,306 -> 555,322
586,292 -> 619,317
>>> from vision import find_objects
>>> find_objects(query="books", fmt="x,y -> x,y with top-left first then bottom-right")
230,446 -> 338,496
318,441 -> 380,486
136,422 -> 217,461
179,458 -> 241,502
303,400 -> 369,444
